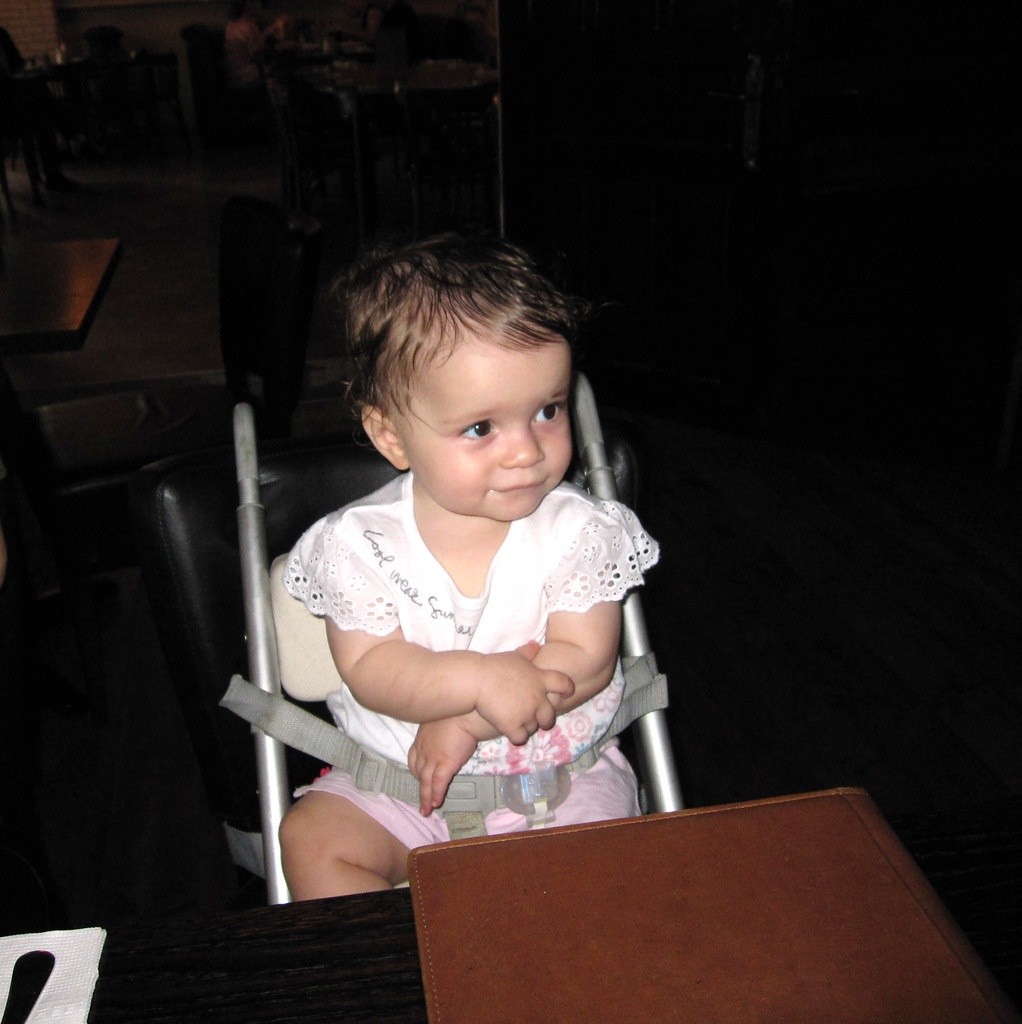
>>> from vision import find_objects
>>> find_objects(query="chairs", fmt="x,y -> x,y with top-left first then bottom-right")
91,25 -> 188,158
151,358 -> 687,906
181,22 -> 276,153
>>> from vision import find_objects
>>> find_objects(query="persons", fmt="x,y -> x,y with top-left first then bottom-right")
225,0 -> 502,154
56,40 -> 83,66
277,213 -> 662,913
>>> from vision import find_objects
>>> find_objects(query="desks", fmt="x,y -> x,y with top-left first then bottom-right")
292,57 -> 501,248
0,237 -> 126,730
0,787 -> 1022,1024
12,55 -> 89,161
